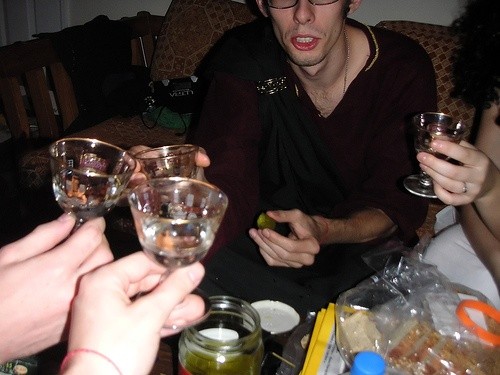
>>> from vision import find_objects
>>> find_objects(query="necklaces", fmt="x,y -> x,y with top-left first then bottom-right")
343,28 -> 350,96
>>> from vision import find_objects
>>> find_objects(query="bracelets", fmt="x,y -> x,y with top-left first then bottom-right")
61,349 -> 123,375
318,216 -> 329,245
456,300 -> 500,345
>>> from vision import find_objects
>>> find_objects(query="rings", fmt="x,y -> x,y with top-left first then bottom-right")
463,181 -> 467,193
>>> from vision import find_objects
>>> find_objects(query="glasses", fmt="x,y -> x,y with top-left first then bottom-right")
266,0 -> 338,9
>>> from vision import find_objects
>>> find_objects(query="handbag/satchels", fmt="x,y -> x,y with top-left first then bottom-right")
154,76 -> 200,113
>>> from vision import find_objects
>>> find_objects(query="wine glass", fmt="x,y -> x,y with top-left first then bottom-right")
49,137 -> 137,235
403,111 -> 471,199
129,177 -> 228,330
133,144 -> 199,180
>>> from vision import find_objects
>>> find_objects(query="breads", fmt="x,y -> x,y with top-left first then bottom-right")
339,312 -> 488,375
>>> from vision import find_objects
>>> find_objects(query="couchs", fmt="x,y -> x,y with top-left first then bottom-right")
14,0 -> 483,239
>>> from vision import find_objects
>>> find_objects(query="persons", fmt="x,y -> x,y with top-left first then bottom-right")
0,213 -> 114,364
120,0 -> 437,375
417,0 -> 500,348
59,250 -> 205,375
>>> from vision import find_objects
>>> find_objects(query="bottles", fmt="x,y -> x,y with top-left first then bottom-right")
338,351 -> 386,375
